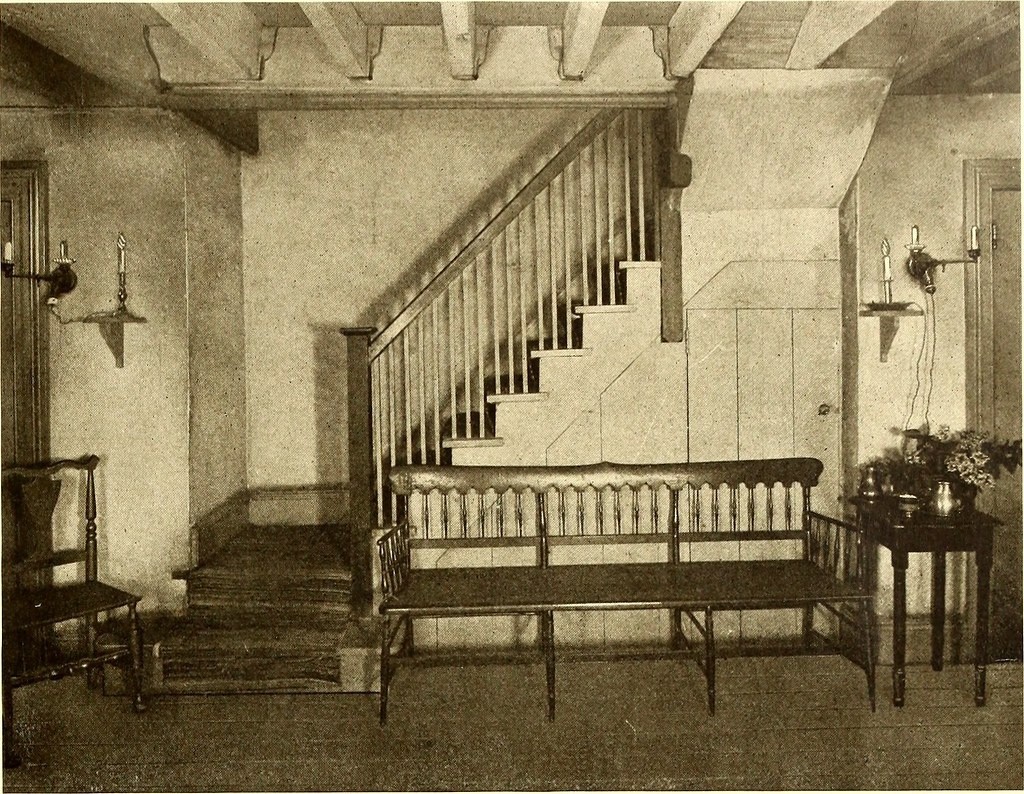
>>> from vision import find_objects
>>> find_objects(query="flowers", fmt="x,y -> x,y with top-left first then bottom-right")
906,425 -> 997,490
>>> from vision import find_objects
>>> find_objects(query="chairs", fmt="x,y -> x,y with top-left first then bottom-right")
2,454 -> 148,771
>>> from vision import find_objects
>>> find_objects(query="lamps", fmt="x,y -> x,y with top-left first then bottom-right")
116,231 -> 127,312
904,225 -> 981,294
1,231 -> 77,307
880,237 -> 893,304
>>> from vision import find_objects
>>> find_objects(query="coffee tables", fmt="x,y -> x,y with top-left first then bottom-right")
848,494 -> 1005,707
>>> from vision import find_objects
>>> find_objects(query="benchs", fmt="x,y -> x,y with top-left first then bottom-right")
375,456 -> 879,723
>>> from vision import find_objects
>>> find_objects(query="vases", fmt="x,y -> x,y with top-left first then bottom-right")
929,481 -> 956,517
858,466 -> 884,508
